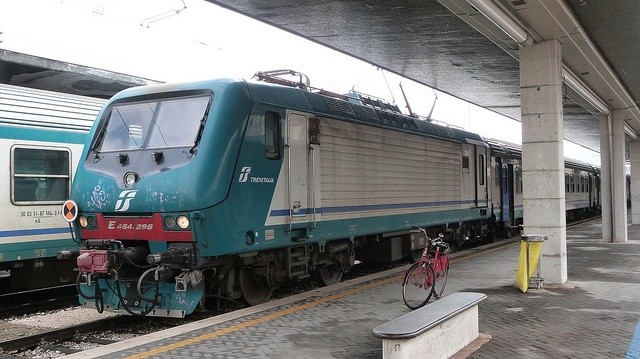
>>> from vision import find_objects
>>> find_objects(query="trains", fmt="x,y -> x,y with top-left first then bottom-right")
0,83 -> 108,284
63,70 -> 630,318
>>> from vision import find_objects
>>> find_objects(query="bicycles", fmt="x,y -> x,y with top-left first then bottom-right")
402,226 -> 451,310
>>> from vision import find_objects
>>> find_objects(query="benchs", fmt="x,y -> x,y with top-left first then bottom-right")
372,291 -> 493,358
